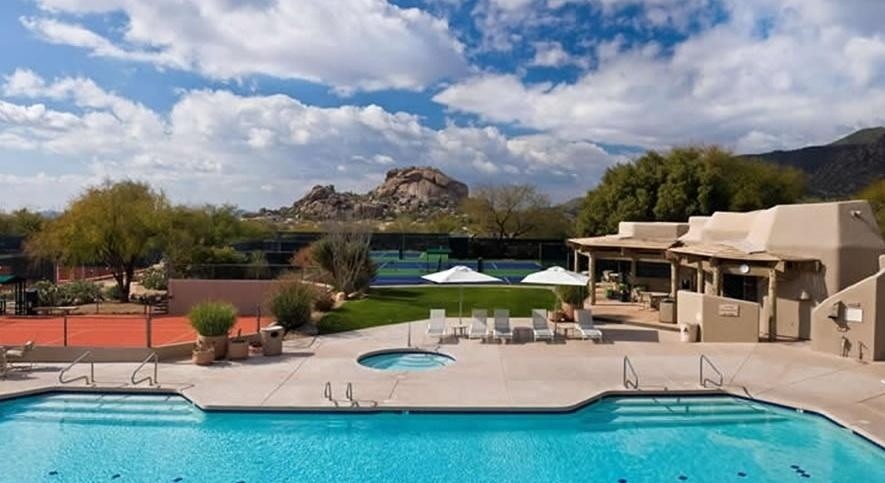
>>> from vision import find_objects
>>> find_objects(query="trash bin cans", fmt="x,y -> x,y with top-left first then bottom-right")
681,322 -> 698,342
660,298 -> 678,322
260,325 -> 285,356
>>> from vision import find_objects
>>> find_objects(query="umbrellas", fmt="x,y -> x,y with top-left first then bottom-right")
420,264 -> 504,324
518,265 -> 593,331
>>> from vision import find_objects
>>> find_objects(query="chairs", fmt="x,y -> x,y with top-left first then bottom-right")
424,304 -> 605,344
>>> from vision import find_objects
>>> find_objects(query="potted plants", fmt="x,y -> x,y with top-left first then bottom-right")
555,285 -> 590,321
186,300 -> 249,367
